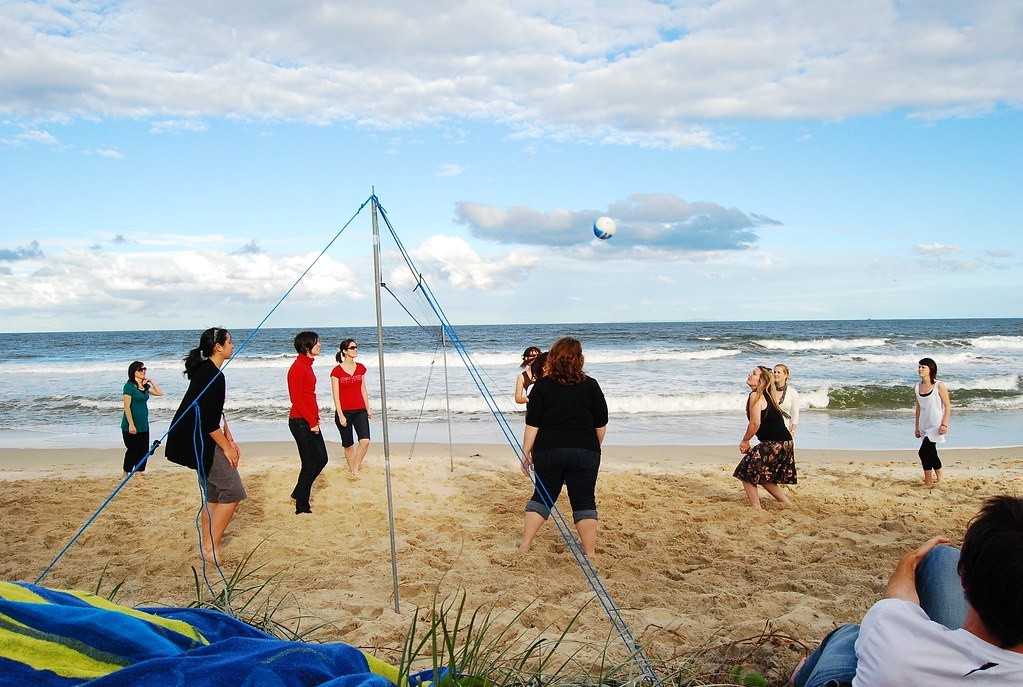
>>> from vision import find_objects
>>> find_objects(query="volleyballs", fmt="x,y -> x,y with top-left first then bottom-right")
593,216 -> 617,241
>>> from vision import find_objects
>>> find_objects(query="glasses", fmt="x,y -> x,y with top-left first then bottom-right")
347,346 -> 357,350
213,326 -> 220,342
136,367 -> 146,372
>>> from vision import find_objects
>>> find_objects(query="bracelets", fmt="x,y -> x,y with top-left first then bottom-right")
791,430 -> 795,432
942,424 -> 947,427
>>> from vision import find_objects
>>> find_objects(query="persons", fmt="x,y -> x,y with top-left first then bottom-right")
790,495 -> 1023,687
915,358 -> 950,486
515,347 -> 541,404
330,339 -> 373,476
527,352 -> 551,404
287,332 -> 328,515
121,361 -> 163,477
165,328 -> 247,565
521,336 -> 608,555
774,364 -> 799,437
732,366 -> 797,509
